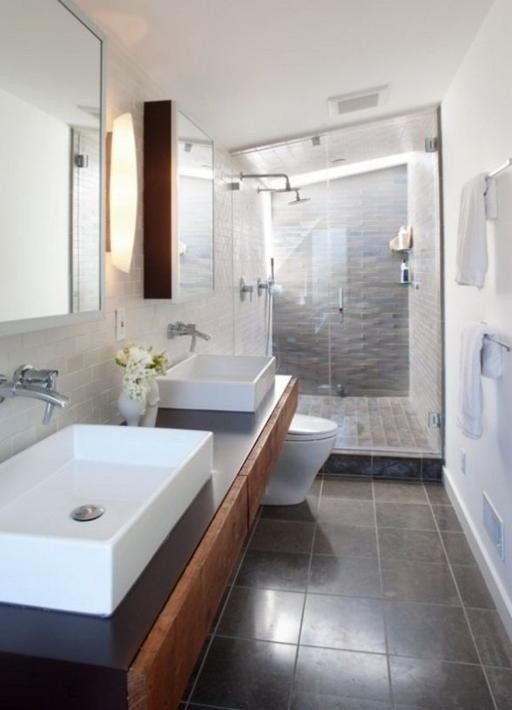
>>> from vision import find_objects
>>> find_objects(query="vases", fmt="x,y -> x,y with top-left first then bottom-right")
118,374 -> 160,427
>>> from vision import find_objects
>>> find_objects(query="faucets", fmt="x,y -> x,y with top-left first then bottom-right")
168,322 -> 210,352
1,376 -> 69,424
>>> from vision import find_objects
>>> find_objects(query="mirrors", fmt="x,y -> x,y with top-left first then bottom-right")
2,1 -> 106,339
173,136 -> 216,306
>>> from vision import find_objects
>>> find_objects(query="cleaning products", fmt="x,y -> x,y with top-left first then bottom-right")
401,257 -> 410,282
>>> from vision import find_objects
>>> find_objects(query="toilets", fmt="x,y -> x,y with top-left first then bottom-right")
260,414 -> 338,505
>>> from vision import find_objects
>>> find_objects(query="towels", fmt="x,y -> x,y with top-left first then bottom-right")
449,170 -> 502,440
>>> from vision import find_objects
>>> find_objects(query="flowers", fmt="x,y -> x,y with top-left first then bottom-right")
116,345 -> 169,403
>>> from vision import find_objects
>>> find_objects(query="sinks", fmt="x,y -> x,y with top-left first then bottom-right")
155,354 -> 277,412
1,423 -> 213,619
